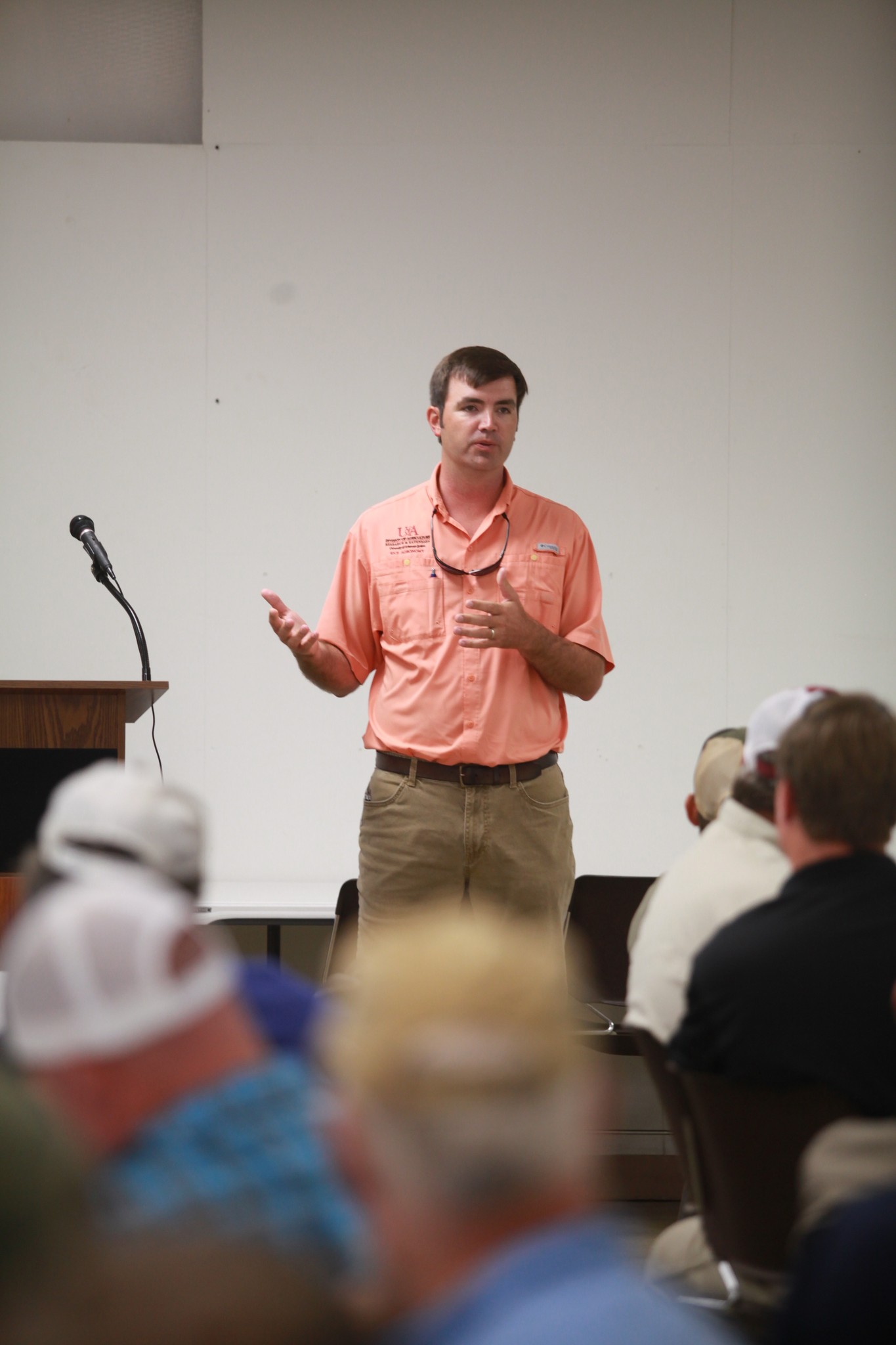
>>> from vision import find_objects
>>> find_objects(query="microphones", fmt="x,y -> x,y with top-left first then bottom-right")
70,515 -> 116,580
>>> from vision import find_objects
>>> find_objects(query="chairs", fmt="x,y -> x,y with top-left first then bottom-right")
564,875 -> 658,1057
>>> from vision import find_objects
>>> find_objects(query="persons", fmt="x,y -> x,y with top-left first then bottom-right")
626,725 -> 750,955
258,344 -> 618,1010
299,901 -> 741,1345
622,681 -> 842,1045
1,875 -> 385,1345
667,695 -> 896,1345
1,762 -> 346,1059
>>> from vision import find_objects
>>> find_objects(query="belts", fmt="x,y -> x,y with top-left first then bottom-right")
374,716 -> 563,788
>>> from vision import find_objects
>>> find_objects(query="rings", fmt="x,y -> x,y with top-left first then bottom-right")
491,627 -> 495,639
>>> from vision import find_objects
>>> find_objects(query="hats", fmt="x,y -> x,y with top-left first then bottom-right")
744,686 -> 841,778
693,724 -> 747,822
34,763 -> 205,895
0,869 -> 242,1066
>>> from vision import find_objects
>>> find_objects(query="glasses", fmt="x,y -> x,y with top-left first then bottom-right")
430,505 -> 509,576
